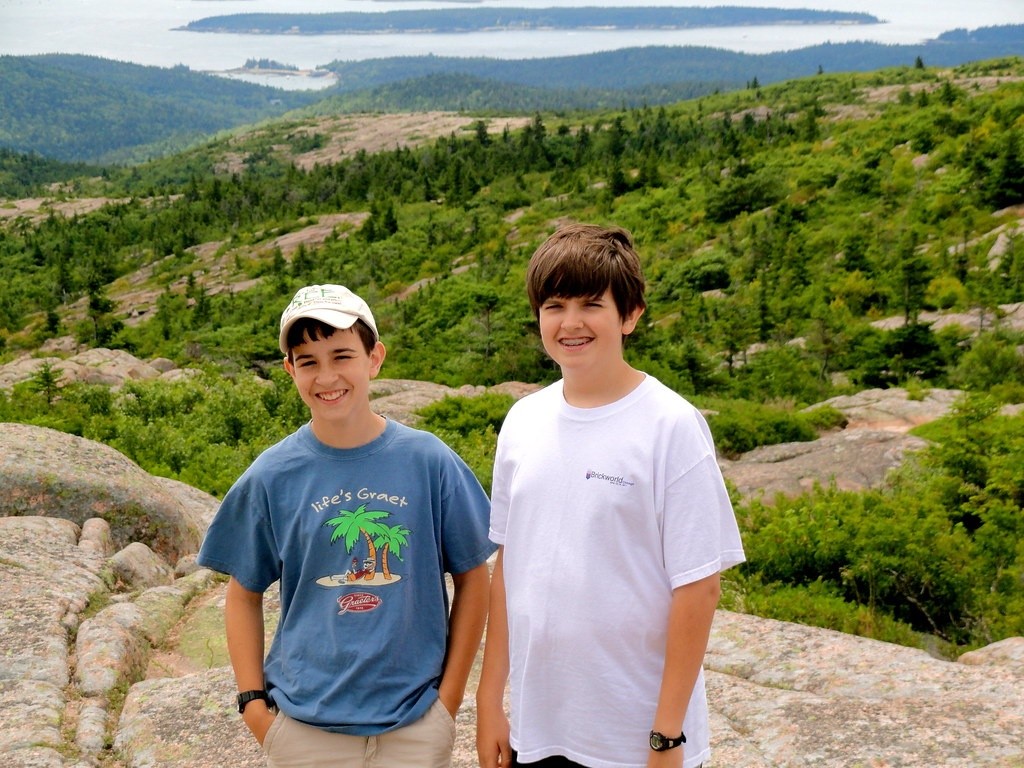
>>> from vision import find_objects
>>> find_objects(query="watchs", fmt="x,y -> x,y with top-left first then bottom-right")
649,729 -> 688,751
233,689 -> 272,714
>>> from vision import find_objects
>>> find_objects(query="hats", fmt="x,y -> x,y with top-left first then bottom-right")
276,283 -> 383,355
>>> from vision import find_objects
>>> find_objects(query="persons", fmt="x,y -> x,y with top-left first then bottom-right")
196,285 -> 496,768
476,218 -> 747,767
